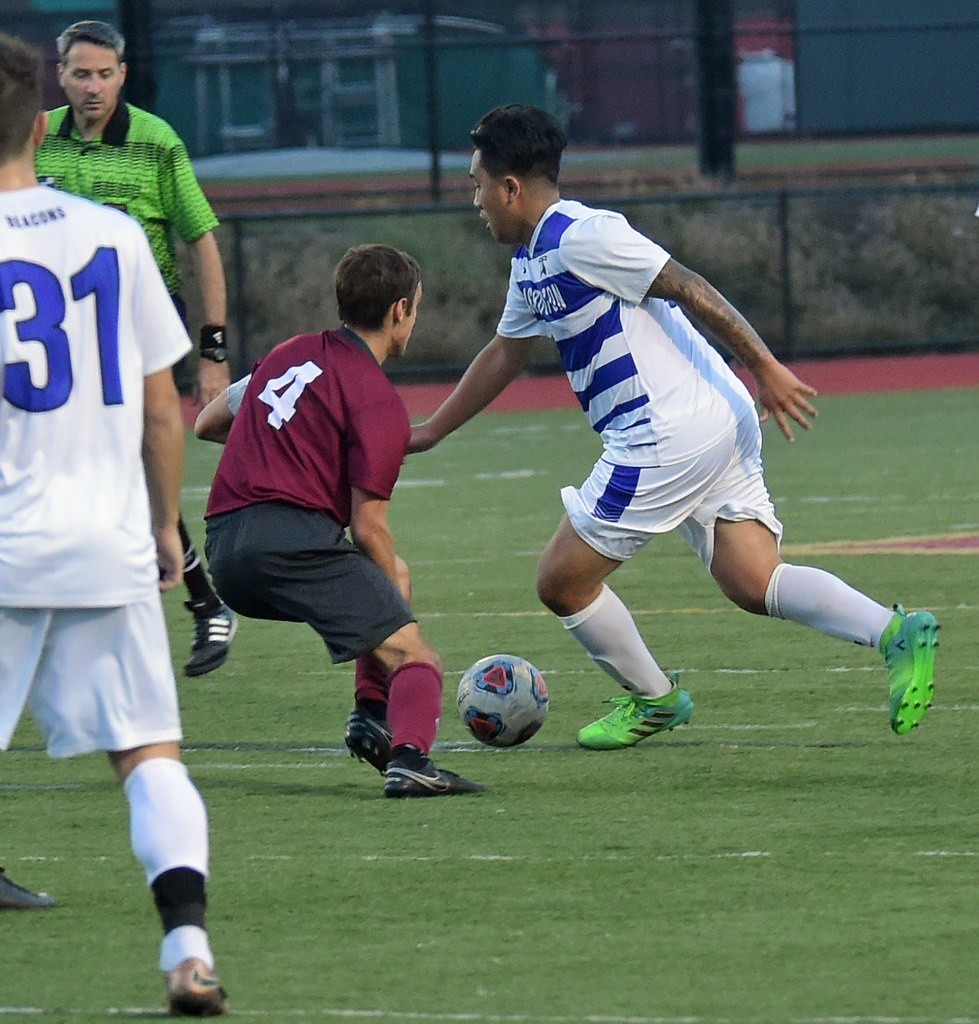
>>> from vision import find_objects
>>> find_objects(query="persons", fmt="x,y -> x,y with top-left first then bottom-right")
404,102 -> 942,752
194,244 -> 491,799
0,32 -> 231,1018
33,20 -> 232,679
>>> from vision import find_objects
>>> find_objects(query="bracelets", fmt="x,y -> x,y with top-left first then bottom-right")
200,324 -> 229,348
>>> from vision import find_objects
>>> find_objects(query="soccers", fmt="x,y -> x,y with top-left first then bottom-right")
452,653 -> 554,749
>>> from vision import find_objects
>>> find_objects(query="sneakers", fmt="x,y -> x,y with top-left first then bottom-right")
182,593 -> 238,677
882,603 -> 942,736
0,868 -> 55,909
343,711 -> 398,775
164,960 -> 229,1013
384,748 -> 487,798
577,678 -> 694,749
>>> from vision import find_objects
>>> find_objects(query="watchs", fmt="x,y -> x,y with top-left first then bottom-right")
199,347 -> 229,363
